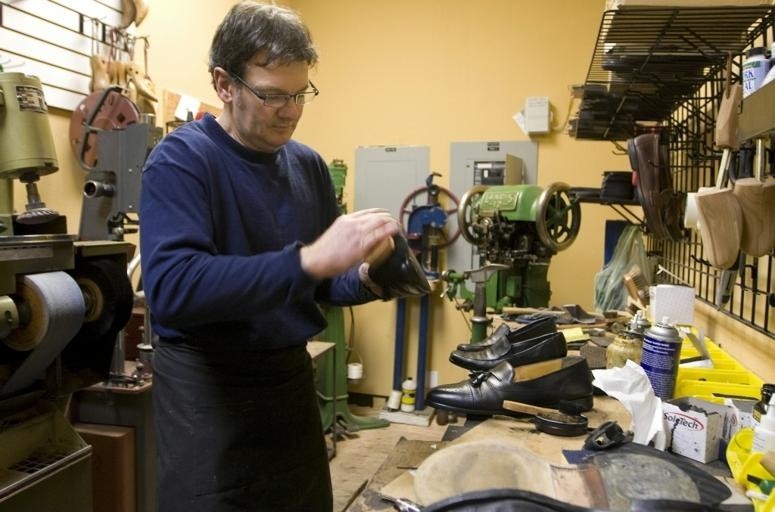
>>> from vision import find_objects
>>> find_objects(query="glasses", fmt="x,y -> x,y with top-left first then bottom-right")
231,71 -> 319,106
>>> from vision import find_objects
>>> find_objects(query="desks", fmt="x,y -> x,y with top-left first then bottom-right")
304,342 -> 336,461
443,414 -> 488,440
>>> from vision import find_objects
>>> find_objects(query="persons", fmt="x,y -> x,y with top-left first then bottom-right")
138,2 -> 436,512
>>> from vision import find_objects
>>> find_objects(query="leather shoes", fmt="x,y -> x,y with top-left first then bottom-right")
451,331 -> 568,371
627,132 -> 685,241
367,228 -> 432,301
424,357 -> 595,416
451,316 -> 557,351
